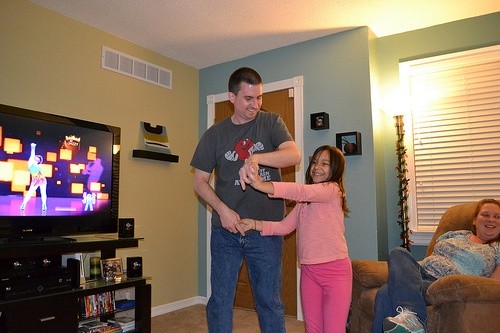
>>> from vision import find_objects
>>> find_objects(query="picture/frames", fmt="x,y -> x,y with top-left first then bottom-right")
100,258 -> 123,277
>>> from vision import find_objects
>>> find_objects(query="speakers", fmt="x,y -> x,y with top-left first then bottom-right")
118,217 -> 134,238
126,257 -> 142,278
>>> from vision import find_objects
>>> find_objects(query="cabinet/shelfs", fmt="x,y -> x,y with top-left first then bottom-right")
0,233 -> 152,333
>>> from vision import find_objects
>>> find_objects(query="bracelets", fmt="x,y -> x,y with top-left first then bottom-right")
254,219 -> 256,230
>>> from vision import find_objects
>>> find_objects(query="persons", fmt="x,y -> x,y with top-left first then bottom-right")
372,199 -> 500,333
111,262 -> 121,272
190,67 -> 302,333
20,144 -> 47,211
239,145 -> 353,333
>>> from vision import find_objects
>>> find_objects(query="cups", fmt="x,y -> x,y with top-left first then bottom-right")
90,257 -> 101,280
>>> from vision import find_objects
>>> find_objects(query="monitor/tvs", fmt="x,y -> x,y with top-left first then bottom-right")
0,104 -> 121,242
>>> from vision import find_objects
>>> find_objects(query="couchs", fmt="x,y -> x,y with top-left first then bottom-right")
347,202 -> 500,333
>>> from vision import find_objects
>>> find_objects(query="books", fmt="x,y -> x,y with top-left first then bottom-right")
82,290 -> 116,318
79,317 -> 135,333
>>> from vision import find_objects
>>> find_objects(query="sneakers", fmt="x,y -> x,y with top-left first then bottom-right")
383,305 -> 426,333
